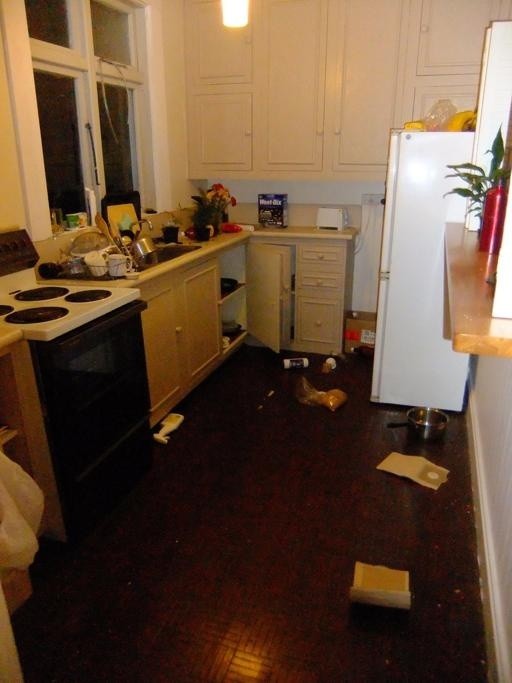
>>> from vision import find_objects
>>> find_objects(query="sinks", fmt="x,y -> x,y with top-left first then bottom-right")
135,245 -> 202,264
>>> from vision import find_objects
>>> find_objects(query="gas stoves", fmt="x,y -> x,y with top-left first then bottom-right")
1,283 -> 139,339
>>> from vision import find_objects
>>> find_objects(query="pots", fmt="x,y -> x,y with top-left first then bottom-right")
387,405 -> 449,441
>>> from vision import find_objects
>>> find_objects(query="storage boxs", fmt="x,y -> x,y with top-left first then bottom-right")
343,309 -> 377,355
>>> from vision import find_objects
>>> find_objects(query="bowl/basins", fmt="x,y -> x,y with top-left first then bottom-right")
130,234 -> 159,262
223,323 -> 242,335
222,276 -> 239,289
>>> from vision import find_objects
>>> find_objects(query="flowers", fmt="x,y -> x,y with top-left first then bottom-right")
190,184 -> 236,227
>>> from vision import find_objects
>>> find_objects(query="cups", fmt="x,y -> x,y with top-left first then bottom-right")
161,225 -> 179,242
61,255 -> 86,279
108,254 -> 133,277
84,250 -> 109,277
124,272 -> 139,279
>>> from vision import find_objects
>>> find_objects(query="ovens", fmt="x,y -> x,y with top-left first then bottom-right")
23,301 -> 167,545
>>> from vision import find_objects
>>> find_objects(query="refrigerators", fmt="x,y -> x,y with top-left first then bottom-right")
369,129 -> 474,413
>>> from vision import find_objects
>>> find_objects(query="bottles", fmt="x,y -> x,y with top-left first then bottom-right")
478,172 -> 506,254
51,207 -> 87,235
222,223 -> 242,232
180,222 -> 218,240
238,222 -> 255,231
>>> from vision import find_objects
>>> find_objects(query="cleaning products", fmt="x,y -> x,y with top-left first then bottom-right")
153,413 -> 184,444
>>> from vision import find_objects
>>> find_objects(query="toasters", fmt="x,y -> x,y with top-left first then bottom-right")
312,205 -> 349,231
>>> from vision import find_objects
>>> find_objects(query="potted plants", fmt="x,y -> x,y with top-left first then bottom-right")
443,122 -> 510,240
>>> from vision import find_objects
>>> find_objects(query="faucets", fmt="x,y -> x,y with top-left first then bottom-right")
129,219 -> 154,242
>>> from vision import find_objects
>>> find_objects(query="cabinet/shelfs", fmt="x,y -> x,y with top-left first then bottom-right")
216,241 -> 294,356
294,242 -> 355,356
137,255 -> 221,412
